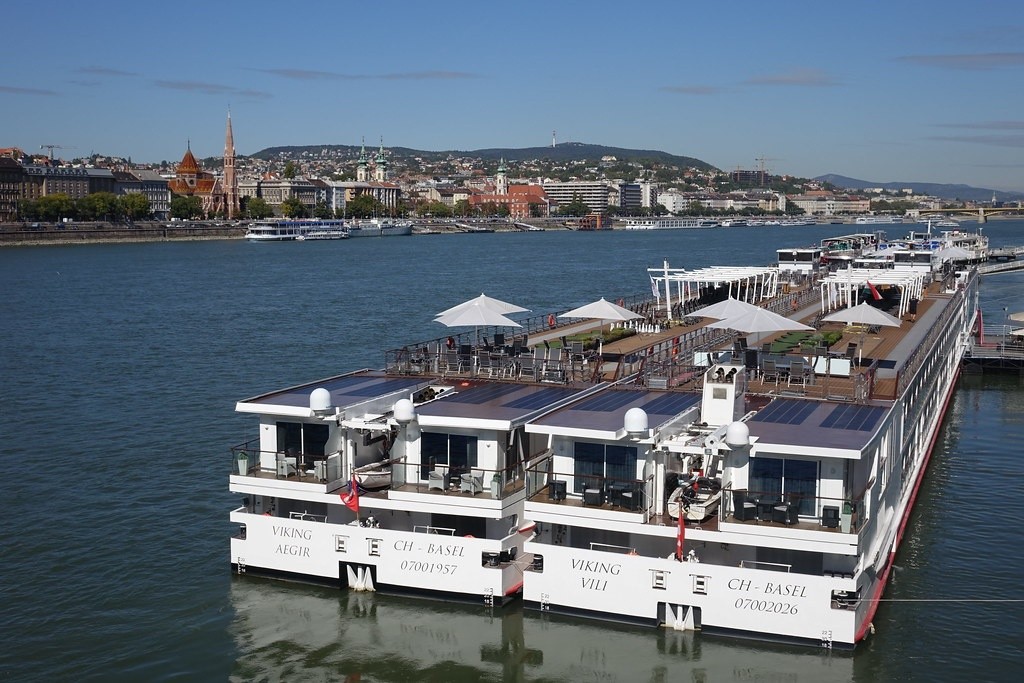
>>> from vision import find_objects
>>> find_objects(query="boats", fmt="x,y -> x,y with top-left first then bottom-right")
245,219 -> 351,242
349,216 -> 414,236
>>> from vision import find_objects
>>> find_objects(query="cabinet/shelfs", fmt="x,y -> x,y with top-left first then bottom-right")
548,480 -> 566,502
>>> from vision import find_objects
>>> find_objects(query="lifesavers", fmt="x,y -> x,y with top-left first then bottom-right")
548,314 -> 555,326
619,299 -> 624,307
447,337 -> 456,350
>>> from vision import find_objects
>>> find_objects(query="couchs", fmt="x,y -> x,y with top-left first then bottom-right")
732,488 -> 758,522
775,491 -> 803,525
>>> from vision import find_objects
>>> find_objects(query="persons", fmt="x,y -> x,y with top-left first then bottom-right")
684,296 -> 818,379
825,239 -> 914,251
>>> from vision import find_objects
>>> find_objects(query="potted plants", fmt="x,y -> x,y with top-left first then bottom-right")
237,450 -> 249,475
491,473 -> 502,499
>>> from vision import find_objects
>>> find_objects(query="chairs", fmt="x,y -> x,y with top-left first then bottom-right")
413,282 -> 735,386
745,348 -> 760,382
788,361 -> 806,388
815,347 -> 827,355
459,467 -> 485,496
427,463 -> 450,492
581,473 -> 605,507
737,337 -> 748,348
762,342 -> 773,355
747,268 -> 814,306
761,359 -> 781,387
819,340 -> 830,351
314,461 -> 326,482
734,340 -> 742,354
621,479 -> 643,512
275,450 -> 297,479
730,357 -> 744,365
841,343 -> 858,371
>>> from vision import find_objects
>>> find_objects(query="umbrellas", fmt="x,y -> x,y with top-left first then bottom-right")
556,296 -> 647,358
433,293 -> 537,352
820,301 -> 903,371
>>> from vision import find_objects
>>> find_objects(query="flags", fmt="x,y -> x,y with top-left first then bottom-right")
862,280 -> 883,300
339,474 -> 359,512
677,502 -> 686,560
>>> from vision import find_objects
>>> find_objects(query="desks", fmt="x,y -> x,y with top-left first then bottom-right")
298,463 -> 307,477
759,500 -> 778,522
608,485 -> 624,507
450,477 -> 459,492
718,352 -> 809,383
803,350 -> 846,359
